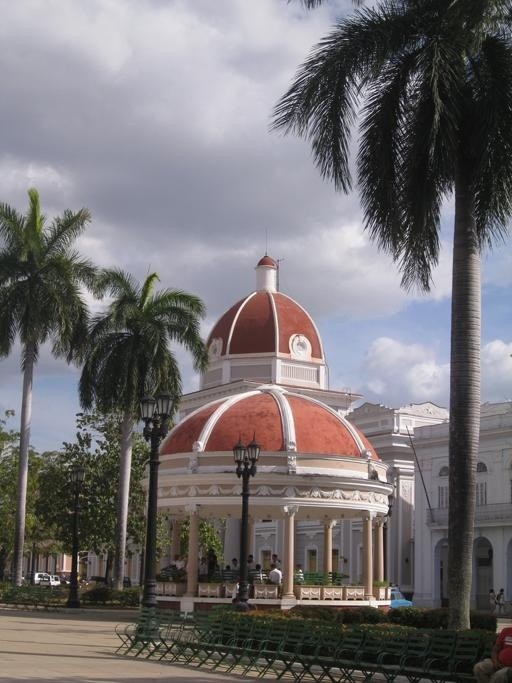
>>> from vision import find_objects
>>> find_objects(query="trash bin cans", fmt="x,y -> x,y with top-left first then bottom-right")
4,575 -> 12,582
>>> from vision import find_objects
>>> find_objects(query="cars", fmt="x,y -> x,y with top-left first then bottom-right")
390,588 -> 412,609
35,572 -> 60,586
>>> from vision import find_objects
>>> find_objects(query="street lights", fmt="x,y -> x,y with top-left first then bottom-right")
30,534 -> 37,584
233,432 -> 260,602
70,469 -> 85,608
140,391 -> 175,629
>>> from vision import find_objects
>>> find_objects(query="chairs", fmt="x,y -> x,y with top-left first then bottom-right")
115,608 -> 498,682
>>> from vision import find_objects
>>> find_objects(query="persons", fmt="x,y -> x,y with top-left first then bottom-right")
489,588 -> 497,614
496,588 -> 507,615
170,547 -> 304,585
473,626 -> 511,682
